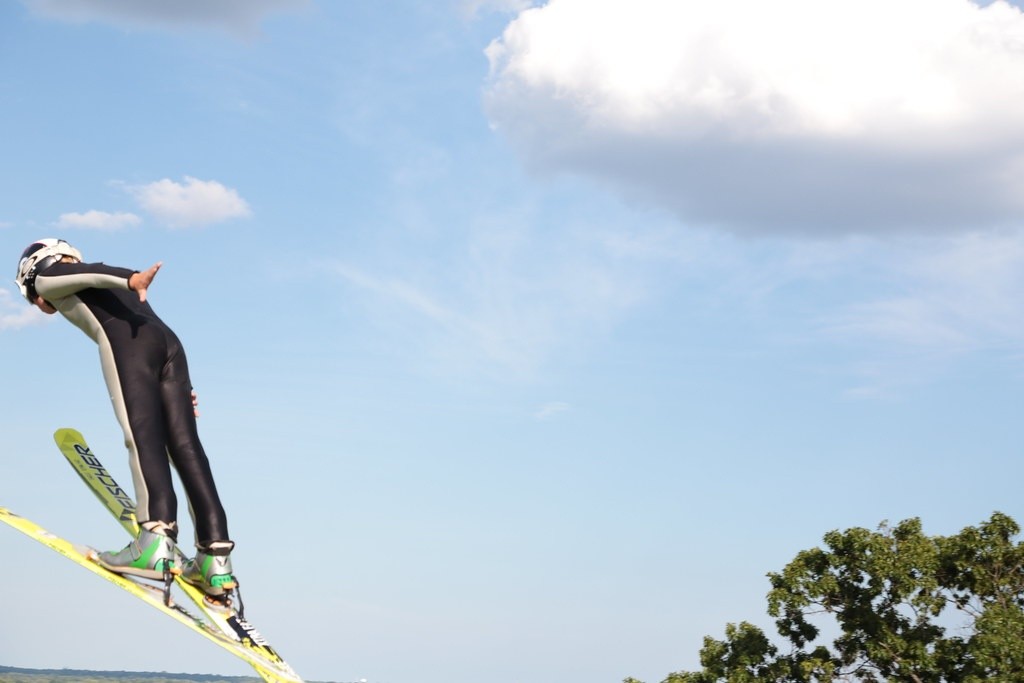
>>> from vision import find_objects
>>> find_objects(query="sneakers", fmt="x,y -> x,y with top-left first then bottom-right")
179,540 -> 236,596
97,525 -> 174,582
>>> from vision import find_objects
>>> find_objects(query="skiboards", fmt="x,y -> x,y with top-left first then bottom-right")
0,427 -> 306,683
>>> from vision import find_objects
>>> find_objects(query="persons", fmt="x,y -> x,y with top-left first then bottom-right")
15,237 -> 240,601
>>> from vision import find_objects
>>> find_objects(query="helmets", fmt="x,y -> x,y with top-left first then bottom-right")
14,238 -> 81,305
